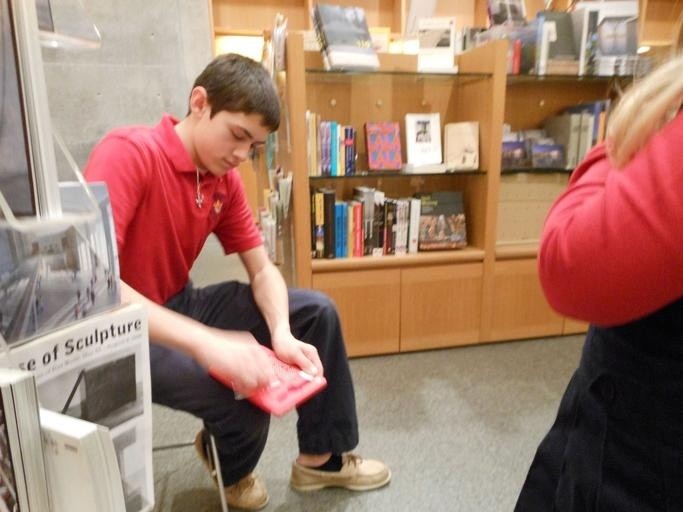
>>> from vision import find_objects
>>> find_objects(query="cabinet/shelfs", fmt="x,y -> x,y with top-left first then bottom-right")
208,0 -> 683,358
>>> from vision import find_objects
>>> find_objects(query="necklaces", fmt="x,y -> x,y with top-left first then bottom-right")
195,166 -> 205,209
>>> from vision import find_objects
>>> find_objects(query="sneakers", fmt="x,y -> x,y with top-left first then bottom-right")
194,428 -> 269,511
288,453 -> 392,492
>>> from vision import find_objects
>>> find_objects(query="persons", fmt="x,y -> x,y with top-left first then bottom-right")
513,49 -> 682,511
79,54 -> 390,511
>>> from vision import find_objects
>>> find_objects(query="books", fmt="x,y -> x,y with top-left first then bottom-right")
310,5 -> 380,69
503,97 -> 616,167
0,371 -> 125,512
306,109 -> 480,258
417,18 -> 457,72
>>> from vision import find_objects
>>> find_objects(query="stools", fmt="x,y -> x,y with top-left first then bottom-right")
151,430 -> 232,512
